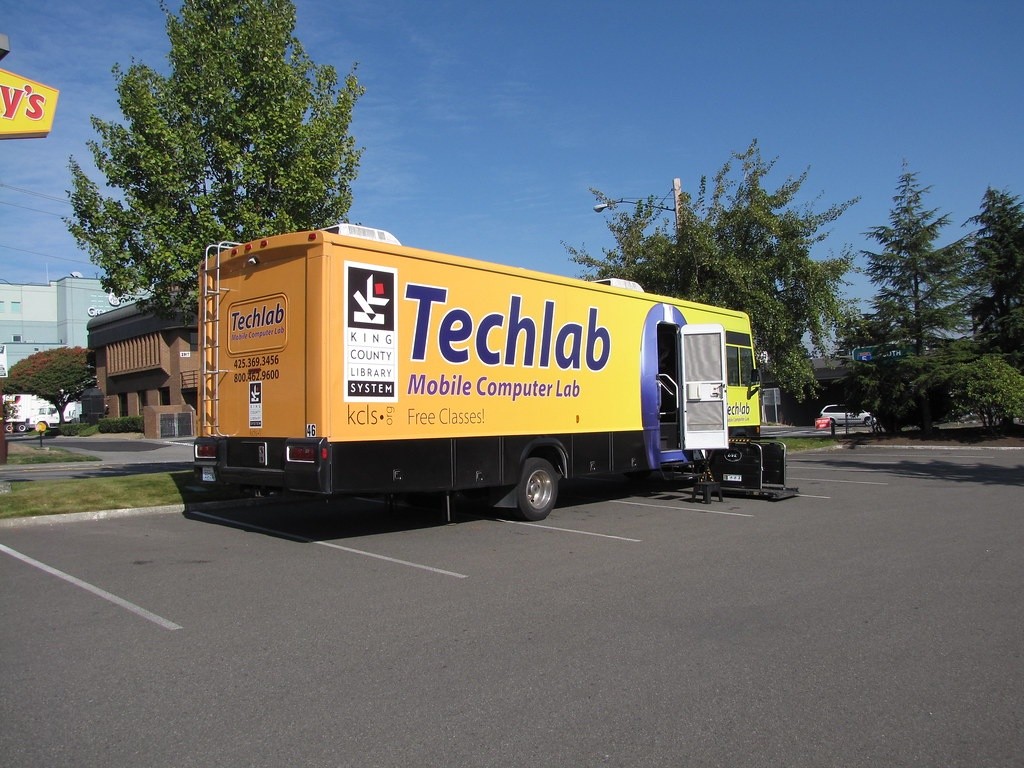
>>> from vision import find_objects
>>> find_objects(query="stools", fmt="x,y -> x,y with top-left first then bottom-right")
692,482 -> 723,504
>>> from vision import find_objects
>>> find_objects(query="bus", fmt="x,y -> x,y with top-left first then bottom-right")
193,229 -> 762,521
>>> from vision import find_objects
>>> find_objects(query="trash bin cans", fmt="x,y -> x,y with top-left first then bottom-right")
0,422 -> 8,463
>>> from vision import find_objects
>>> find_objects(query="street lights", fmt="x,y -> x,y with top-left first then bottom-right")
594,177 -> 684,247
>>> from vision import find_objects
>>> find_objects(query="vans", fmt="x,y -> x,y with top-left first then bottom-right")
819,404 -> 876,427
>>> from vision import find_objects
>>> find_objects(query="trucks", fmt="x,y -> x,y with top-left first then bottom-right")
2,393 -> 61,433
